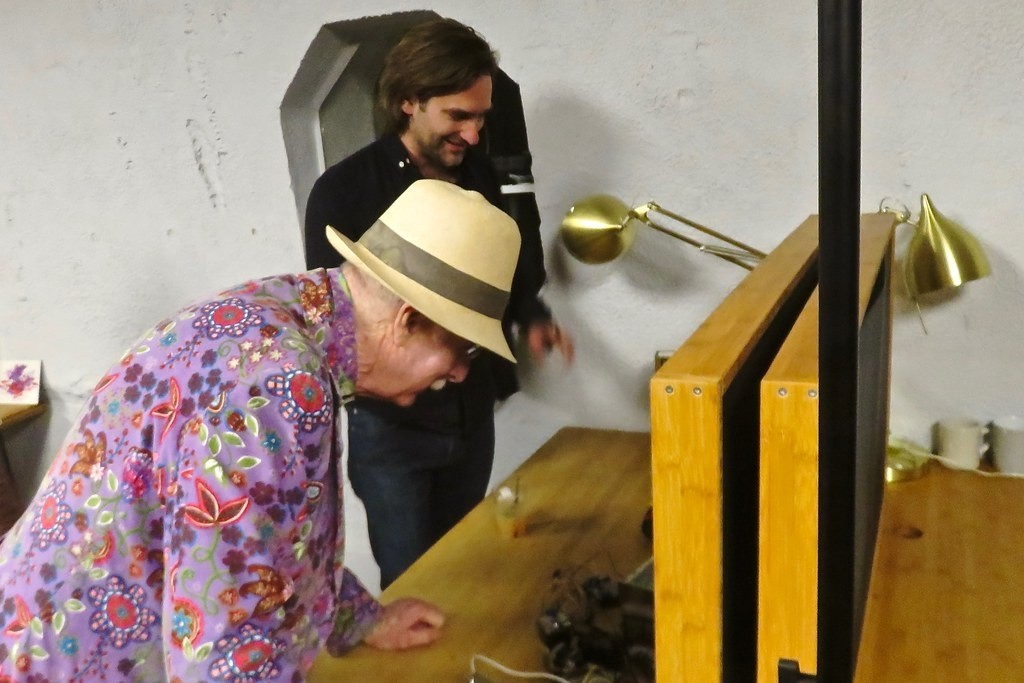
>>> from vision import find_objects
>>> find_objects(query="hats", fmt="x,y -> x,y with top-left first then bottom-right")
325,179 -> 522,364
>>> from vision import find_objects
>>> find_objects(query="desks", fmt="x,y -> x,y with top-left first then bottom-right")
852,453 -> 1024,683
304,427 -> 654,683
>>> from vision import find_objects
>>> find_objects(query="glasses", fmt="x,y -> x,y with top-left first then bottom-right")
440,326 -> 483,363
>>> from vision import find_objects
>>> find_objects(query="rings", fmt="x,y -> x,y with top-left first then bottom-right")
553,327 -> 559,334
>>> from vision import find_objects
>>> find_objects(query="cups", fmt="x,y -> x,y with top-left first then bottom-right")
993,415 -> 1024,474
938,417 -> 983,469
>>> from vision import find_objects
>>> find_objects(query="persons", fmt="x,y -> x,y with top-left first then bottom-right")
0,179 -> 521,683
304,19 -> 575,592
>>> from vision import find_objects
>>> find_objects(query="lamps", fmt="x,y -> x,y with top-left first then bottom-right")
562,194 -> 767,272
877,182 -> 992,482
